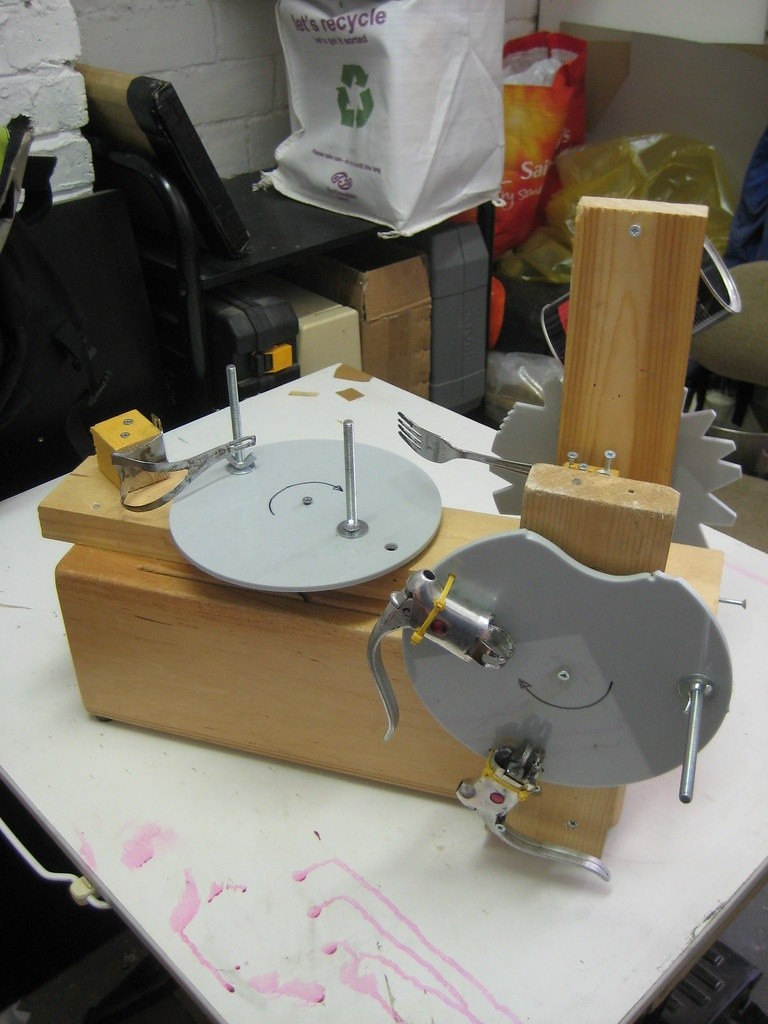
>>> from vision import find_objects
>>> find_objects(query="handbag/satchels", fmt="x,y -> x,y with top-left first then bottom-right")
495,129 -> 734,284
260,0 -> 504,236
493,32 -> 589,255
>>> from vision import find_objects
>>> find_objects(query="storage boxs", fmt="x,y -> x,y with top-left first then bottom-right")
417,221 -> 489,414
149,285 -> 298,421
317,250 -> 432,400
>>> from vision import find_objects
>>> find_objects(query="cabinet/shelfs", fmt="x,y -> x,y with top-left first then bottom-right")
106,149 -> 495,411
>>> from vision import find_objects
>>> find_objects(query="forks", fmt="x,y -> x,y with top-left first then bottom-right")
396,411 -> 531,475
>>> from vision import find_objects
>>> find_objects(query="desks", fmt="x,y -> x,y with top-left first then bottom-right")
0,361 -> 768,1024
